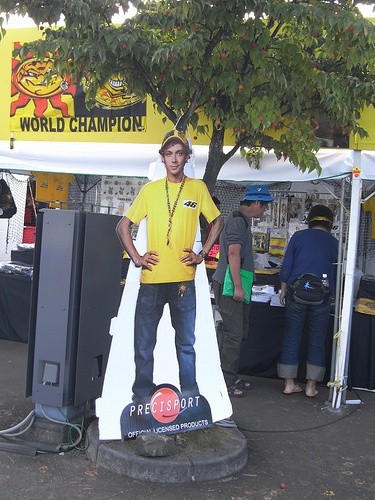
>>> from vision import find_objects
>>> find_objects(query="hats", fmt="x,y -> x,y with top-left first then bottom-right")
240,184 -> 274,202
161,129 -> 188,149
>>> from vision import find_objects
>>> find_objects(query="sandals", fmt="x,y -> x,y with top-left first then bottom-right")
227,378 -> 253,398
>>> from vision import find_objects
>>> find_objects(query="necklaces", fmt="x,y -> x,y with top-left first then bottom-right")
164,175 -> 187,245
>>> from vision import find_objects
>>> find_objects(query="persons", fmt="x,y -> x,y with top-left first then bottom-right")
212,184 -> 274,396
279,204 -> 344,397
199,196 -> 222,247
116,129 -> 224,394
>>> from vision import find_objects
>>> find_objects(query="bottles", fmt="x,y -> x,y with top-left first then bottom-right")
321,274 -> 329,289
256,250 -> 269,270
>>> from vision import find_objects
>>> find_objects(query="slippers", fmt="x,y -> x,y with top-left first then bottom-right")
282,384 -> 303,395
306,389 -> 319,398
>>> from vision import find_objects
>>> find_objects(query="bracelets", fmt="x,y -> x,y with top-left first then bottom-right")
199,249 -> 207,259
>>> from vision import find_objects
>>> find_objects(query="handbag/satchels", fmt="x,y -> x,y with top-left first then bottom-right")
284,273 -> 334,306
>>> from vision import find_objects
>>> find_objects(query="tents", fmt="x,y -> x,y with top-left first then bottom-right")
0,26 -> 375,408
